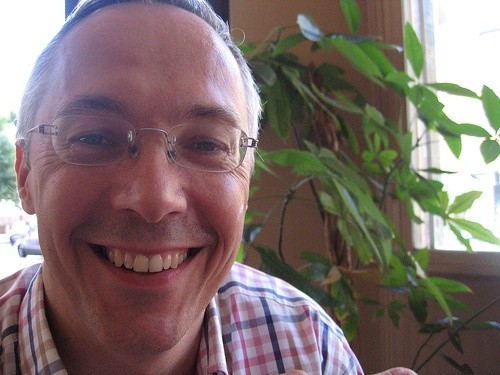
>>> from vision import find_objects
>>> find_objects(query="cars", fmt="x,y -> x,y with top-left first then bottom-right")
9,231 -> 43,258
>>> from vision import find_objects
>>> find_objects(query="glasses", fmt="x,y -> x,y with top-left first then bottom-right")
22,115 -> 261,174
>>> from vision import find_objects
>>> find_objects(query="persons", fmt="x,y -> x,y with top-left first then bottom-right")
0,0 -> 420,375
